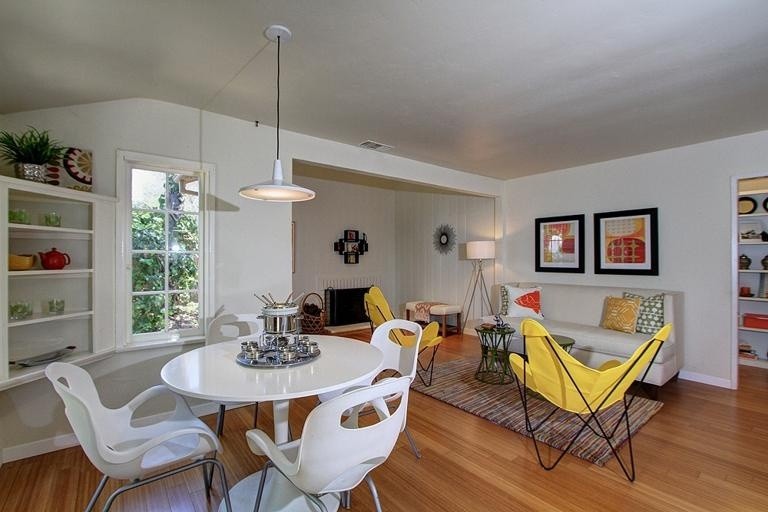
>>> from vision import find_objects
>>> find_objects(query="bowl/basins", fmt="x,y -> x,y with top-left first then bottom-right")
8,253 -> 35,271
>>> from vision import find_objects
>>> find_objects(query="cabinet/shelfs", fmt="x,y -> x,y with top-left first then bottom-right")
738,189 -> 768,369
0,176 -> 118,392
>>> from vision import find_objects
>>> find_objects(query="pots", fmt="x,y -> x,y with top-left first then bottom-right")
256,303 -> 304,335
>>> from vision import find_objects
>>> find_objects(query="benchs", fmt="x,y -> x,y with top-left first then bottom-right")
404,301 -> 461,338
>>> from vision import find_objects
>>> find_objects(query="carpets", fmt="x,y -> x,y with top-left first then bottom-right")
410,356 -> 665,467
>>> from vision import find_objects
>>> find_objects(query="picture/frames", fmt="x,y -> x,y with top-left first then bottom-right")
594,207 -> 659,275
334,230 -> 368,264
292,221 -> 296,274
535,214 -> 585,273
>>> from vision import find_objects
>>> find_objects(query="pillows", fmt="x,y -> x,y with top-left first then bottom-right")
603,296 -> 640,334
623,293 -> 664,336
505,285 -> 542,319
500,287 -> 508,316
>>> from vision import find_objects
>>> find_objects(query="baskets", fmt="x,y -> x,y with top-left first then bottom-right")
301,293 -> 325,334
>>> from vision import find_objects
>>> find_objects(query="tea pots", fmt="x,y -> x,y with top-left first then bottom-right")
37,245 -> 71,270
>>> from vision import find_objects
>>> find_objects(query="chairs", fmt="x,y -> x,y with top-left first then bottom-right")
509,320 -> 671,481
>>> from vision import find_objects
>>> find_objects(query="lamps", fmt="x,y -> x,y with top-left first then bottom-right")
238,24 -> 315,203
461,241 -> 496,335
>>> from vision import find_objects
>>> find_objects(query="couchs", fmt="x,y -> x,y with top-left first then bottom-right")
480,283 -> 683,399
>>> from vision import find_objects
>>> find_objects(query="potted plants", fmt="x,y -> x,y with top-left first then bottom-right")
0,125 -> 66,183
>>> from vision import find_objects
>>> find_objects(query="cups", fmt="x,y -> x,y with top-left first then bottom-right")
46,295 -> 66,314
241,335 -> 320,364
8,208 -> 32,225
7,295 -> 34,321
43,208 -> 62,226
